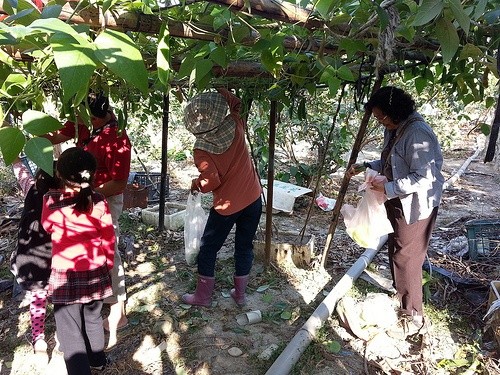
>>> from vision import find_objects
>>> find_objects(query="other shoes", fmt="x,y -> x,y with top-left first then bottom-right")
103,316 -> 129,331
34,339 -> 49,368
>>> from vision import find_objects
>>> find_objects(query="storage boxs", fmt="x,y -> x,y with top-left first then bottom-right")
465,218 -> 500,264
132,171 -> 170,201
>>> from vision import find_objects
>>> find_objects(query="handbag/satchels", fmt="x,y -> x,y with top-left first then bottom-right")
184,190 -> 206,267
340,168 -> 395,250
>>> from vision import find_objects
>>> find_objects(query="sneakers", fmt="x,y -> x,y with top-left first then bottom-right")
387,316 -> 430,337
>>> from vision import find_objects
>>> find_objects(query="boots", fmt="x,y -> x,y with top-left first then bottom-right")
182,273 -> 215,308
230,272 -> 249,305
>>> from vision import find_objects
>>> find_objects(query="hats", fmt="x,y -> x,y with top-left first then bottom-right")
183,92 -> 227,135
74,94 -> 109,118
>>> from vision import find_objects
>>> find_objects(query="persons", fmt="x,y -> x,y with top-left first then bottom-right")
38,87 -> 131,337
40,145 -> 117,375
344,86 -> 446,341
180,82 -> 263,308
8,154 -> 68,368
